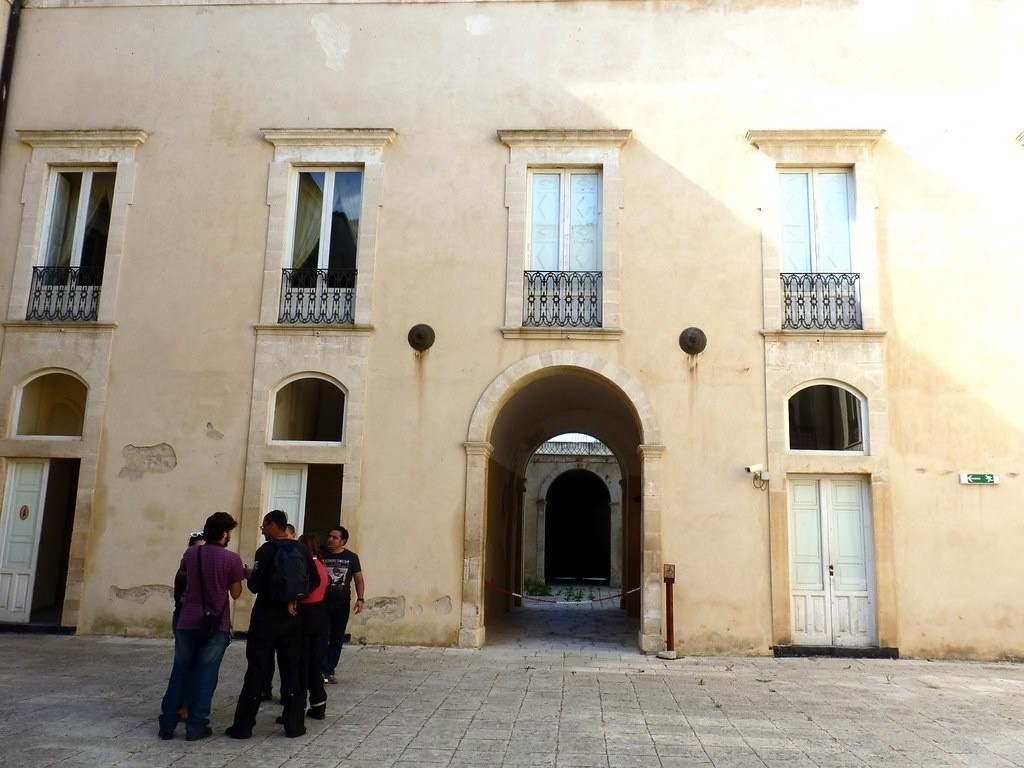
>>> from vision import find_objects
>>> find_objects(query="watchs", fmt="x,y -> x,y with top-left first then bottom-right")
357,598 -> 364,603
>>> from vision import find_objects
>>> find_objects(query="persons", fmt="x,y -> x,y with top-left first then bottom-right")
224,510 -> 328,740
157,512 -> 245,741
318,526 -> 365,684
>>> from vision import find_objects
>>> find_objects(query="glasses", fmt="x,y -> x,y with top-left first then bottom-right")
260,522 -> 272,530
189,532 -> 204,543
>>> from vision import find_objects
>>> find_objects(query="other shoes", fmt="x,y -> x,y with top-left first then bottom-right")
286,727 -> 306,737
261,690 -> 271,700
225,726 -> 252,739
276,716 -> 284,725
178,704 -> 189,721
185,727 -> 212,741
159,728 -> 173,740
321,672 -> 336,684
307,703 -> 326,719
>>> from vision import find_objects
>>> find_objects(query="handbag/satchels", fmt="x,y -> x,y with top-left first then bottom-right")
201,610 -> 221,639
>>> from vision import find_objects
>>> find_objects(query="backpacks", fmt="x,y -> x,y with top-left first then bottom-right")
269,540 -> 309,602
